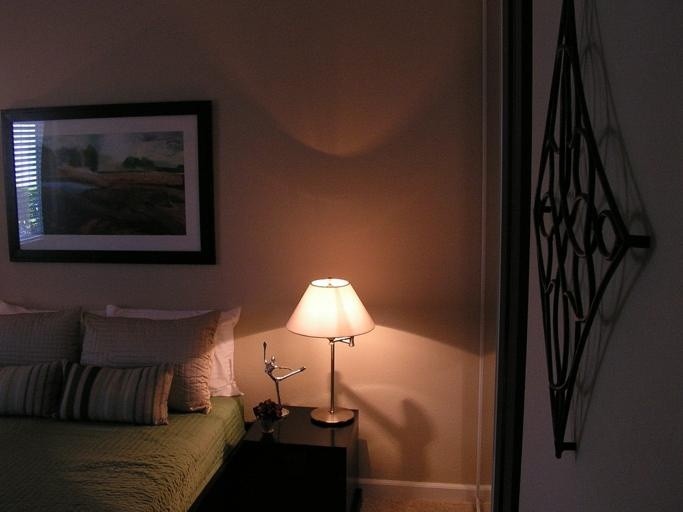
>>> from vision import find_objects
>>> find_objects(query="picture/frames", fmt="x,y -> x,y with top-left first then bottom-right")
1,97 -> 217,265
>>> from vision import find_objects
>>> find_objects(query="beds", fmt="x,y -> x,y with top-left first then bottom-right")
0,395 -> 247,512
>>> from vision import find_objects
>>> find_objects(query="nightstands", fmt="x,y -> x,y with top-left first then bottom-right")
240,402 -> 361,511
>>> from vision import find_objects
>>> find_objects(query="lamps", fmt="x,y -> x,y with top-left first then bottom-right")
284,275 -> 376,428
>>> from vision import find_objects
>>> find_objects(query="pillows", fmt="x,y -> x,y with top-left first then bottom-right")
0,305 -> 86,366
79,311 -> 219,417
0,357 -> 63,419
52,362 -> 175,429
0,298 -> 62,317
103,302 -> 245,397
